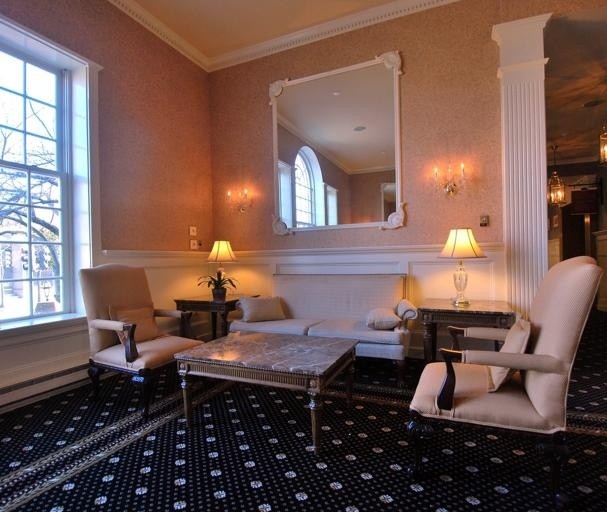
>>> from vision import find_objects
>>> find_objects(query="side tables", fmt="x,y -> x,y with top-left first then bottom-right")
175,292 -> 261,340
419,298 -> 516,363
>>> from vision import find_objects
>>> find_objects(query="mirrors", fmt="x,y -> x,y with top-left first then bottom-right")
268,49 -> 407,237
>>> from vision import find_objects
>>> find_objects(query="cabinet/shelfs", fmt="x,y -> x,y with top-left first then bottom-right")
594,231 -> 607,311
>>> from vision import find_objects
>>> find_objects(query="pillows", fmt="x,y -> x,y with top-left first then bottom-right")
366,307 -> 403,330
240,296 -> 286,322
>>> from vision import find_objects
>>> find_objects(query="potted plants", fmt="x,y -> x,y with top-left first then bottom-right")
196,269 -> 240,301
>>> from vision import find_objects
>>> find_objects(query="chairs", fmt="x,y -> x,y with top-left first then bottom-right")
78,263 -> 204,424
405,255 -> 604,482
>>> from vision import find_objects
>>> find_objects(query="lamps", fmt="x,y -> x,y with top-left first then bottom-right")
547,144 -> 568,207
205,240 -> 239,270
433,161 -> 466,199
437,227 -> 488,308
600,119 -> 607,162
224,188 -> 255,218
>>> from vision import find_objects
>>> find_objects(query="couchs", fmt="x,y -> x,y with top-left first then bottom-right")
229,274 -> 418,387
